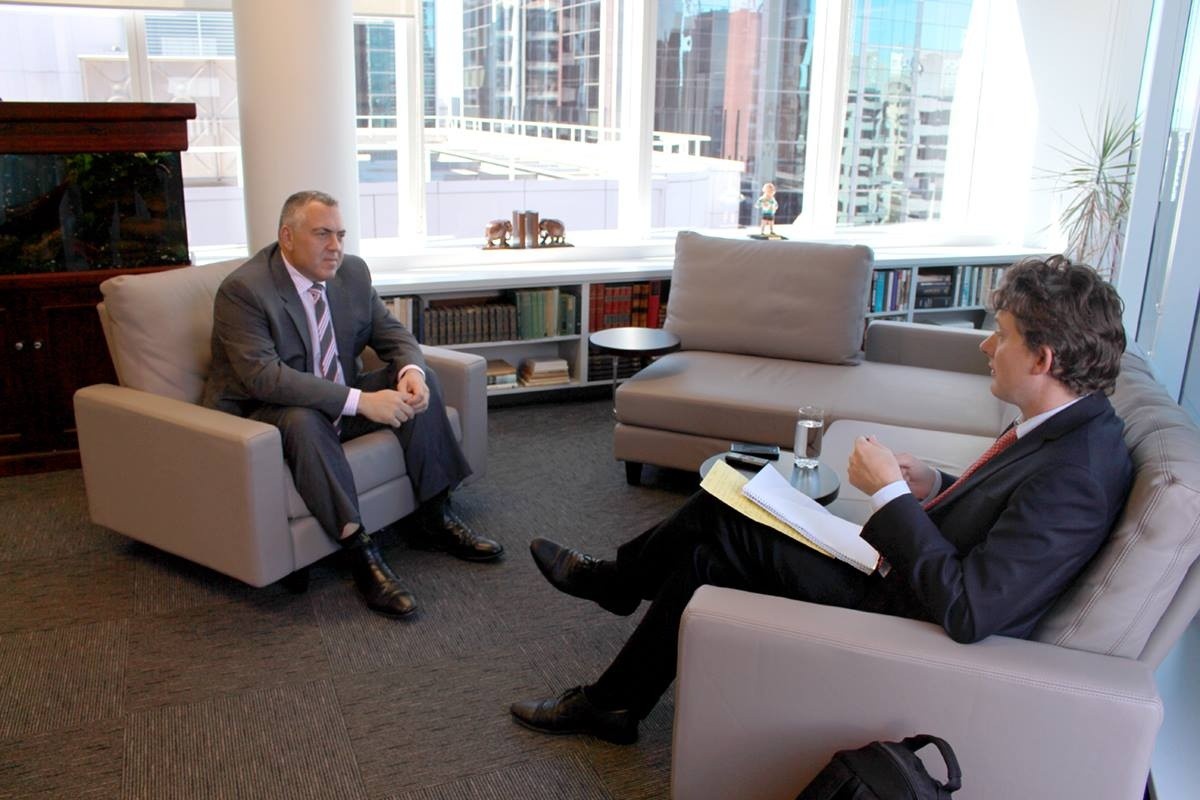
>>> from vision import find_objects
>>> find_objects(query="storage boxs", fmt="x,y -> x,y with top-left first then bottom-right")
0,102 -> 198,281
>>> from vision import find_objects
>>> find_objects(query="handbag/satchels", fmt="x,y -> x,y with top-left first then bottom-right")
794,734 -> 962,800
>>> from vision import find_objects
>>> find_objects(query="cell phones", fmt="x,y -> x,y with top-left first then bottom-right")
726,452 -> 768,471
730,443 -> 780,460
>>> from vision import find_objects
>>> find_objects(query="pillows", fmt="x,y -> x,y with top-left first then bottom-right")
664,230 -> 875,367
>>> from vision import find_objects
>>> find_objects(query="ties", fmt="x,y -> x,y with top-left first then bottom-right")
309,283 -> 345,437
876,422 -> 1021,573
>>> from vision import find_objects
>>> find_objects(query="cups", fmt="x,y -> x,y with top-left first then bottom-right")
794,407 -> 824,469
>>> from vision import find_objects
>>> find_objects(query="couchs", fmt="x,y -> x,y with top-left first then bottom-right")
612,318 -> 1019,486
668,334 -> 1200,800
73,256 -> 487,593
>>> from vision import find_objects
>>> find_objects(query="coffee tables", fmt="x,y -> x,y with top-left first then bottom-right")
697,448 -> 841,510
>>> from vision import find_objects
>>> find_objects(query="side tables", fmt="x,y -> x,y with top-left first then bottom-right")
588,326 -> 682,407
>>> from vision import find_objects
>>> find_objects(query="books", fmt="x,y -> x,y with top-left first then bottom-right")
742,463 -> 880,575
916,265 -> 1004,308
590,283 -> 668,332
589,351 -> 657,381
385,297 -> 424,347
424,288 -> 582,346
861,268 -> 912,351
487,356 -> 570,389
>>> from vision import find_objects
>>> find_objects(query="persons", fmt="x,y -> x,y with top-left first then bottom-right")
203,189 -> 503,623
511,255 -> 1132,744
757,184 -> 778,234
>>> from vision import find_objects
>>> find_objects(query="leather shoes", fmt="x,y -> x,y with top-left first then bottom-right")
530,535 -> 643,616
347,543 -> 419,618
511,682 -> 639,746
408,505 -> 504,561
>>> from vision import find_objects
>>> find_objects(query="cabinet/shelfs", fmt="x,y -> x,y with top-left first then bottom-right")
0,291 -> 119,477
376,240 -> 1058,398
